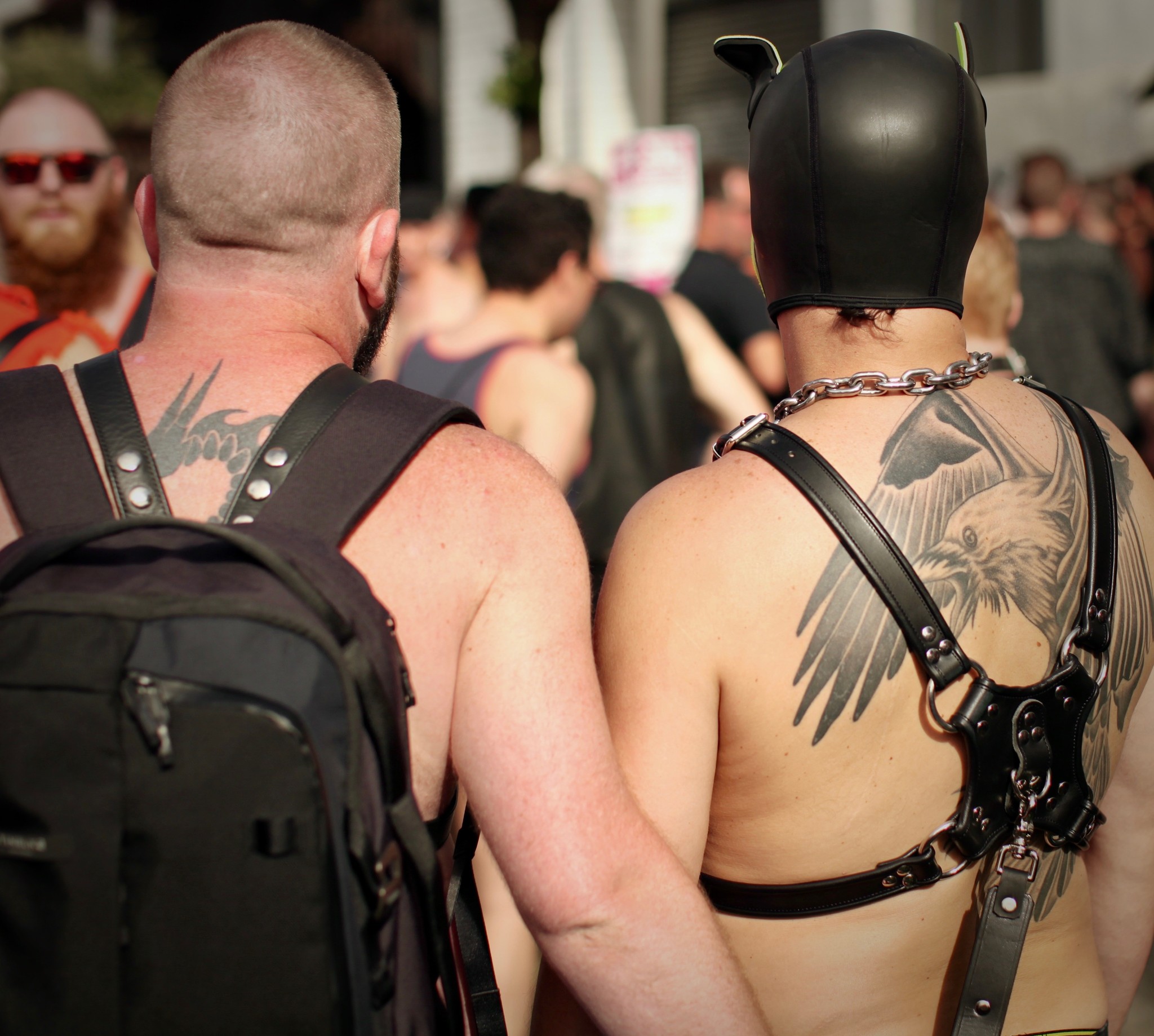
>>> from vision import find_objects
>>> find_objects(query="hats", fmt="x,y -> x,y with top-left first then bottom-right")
710,19 -> 996,320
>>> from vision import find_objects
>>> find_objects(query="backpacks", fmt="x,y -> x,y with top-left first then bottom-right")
0,361 -> 440,1033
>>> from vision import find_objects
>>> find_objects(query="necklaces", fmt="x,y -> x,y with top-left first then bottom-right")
773,349 -> 993,415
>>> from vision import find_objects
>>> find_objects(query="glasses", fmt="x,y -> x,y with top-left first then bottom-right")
1,149 -> 126,186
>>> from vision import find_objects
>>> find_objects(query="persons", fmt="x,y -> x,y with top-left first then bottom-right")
962,202 -> 1029,376
523,157 -> 772,627
397,188 -> 458,277
1,21 -> 775,1036
372,182 -> 594,496
595,20 -> 1154,1036
672,159 -> 785,391
0,86 -> 157,372
1006,151 -> 1154,441
